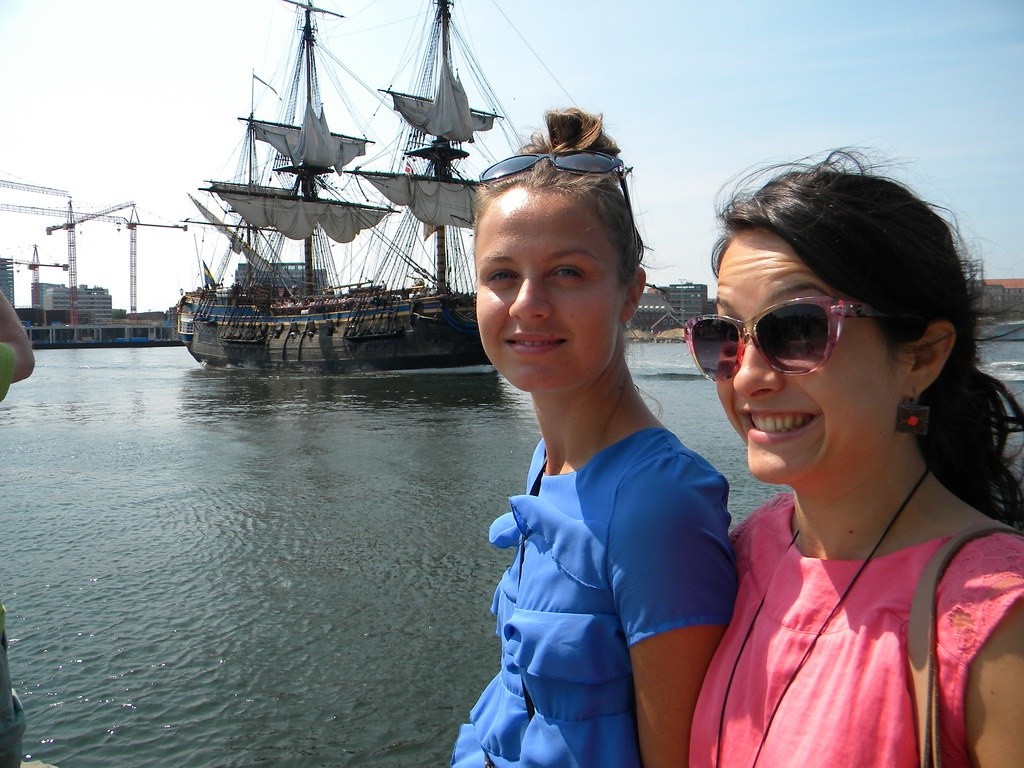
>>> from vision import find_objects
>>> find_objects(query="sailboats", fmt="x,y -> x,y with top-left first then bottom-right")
176,0 -> 503,378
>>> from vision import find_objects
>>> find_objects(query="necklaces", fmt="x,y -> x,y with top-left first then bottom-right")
716,470 -> 928,768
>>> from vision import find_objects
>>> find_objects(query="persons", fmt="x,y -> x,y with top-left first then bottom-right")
688,172 -> 1024,768
0,288 -> 35,768
450,109 -> 738,768
270,280 -> 392,315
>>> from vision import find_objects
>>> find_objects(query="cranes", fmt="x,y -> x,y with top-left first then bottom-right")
128,216 -> 188,314
26,243 -> 71,309
45,196 -> 138,327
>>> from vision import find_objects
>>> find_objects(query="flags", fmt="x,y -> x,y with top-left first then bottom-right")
406,162 -> 410,172
203,261 -> 215,284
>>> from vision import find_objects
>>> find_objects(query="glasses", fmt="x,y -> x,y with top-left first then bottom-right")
684,298 -> 889,381
479,149 -> 640,272
